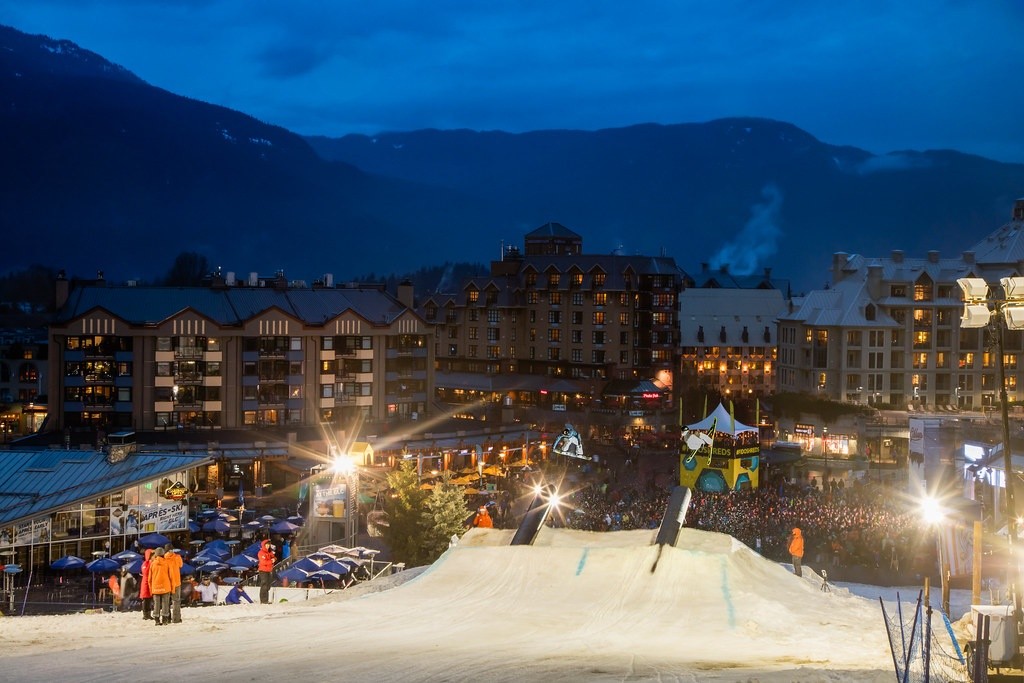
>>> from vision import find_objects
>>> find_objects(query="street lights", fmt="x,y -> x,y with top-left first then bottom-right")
955,276 -> 1023,599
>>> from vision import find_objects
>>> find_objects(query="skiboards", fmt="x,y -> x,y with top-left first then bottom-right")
686,417 -> 717,465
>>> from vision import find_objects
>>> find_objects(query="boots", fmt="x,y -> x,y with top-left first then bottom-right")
143,610 -> 154,620
155,617 -> 161,625
162,615 -> 168,625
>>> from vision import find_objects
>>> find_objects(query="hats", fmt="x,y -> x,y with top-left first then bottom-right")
155,547 -> 165,557
165,544 -> 173,551
479,506 -> 485,511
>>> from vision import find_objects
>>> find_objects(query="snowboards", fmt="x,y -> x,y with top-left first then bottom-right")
552,449 -> 592,461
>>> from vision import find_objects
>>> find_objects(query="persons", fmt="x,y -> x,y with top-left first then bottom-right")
99,539 -> 346,625
472,429 -> 942,585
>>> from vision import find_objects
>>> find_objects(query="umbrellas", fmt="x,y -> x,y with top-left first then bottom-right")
55,510 -> 360,581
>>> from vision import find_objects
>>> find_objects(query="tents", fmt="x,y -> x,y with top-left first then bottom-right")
686,404 -> 759,445
